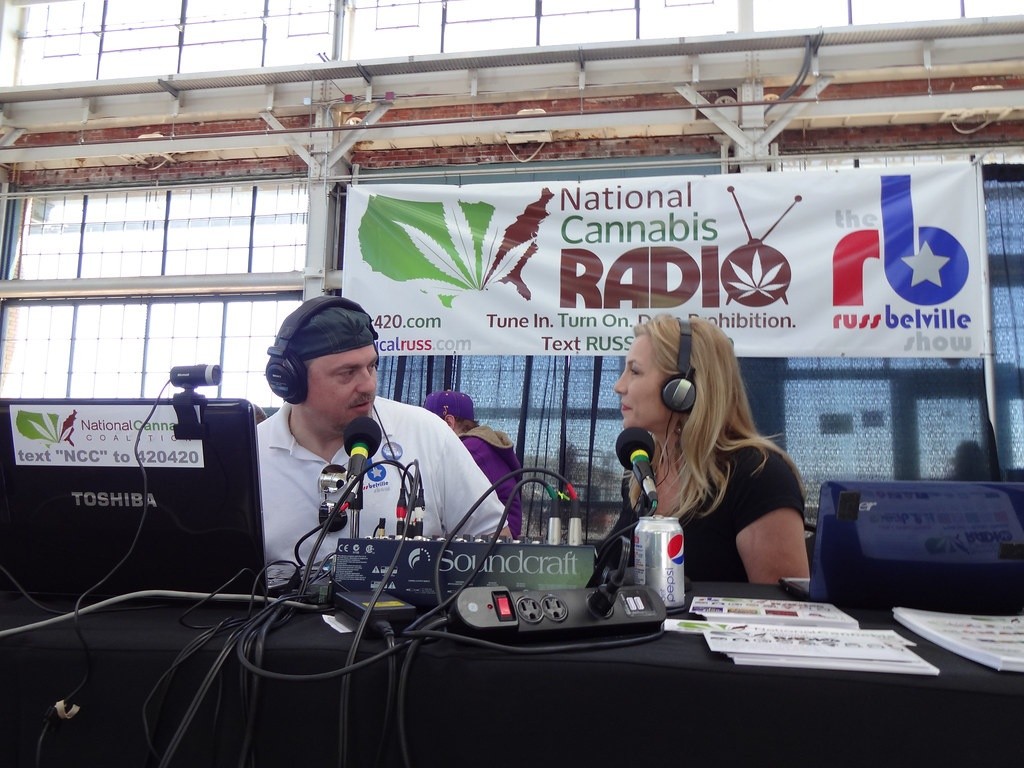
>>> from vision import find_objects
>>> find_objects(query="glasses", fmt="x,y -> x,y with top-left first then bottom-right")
318,464 -> 348,532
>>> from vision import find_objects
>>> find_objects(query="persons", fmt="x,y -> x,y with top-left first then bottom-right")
256,295 -> 522,594
596,312 -> 812,586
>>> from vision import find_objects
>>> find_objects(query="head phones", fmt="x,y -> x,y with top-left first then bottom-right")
661,319 -> 696,413
266,296 -> 380,404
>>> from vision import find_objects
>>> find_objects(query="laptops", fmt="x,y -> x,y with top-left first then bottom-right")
777,482 -> 1024,617
0,398 -> 328,604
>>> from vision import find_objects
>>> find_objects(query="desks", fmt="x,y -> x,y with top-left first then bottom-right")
0,577 -> 1024,768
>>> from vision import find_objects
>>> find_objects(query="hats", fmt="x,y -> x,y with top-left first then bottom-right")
423,389 -> 474,421
273,305 -> 374,361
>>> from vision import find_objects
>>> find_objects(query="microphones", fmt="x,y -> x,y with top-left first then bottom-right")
616,427 -> 658,505
343,416 -> 382,504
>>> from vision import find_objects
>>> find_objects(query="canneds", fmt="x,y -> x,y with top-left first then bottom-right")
634,515 -> 685,610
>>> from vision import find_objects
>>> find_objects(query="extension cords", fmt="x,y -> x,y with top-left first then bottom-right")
449,586 -> 666,651
